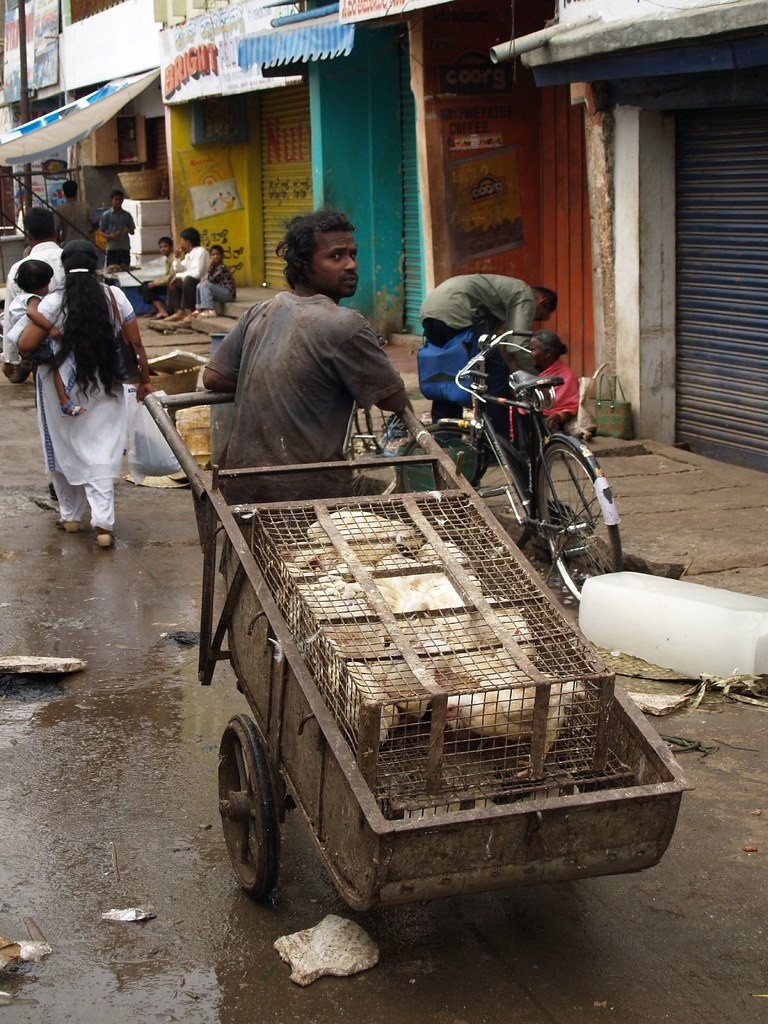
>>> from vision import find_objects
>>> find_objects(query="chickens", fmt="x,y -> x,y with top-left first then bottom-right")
276,506 -> 588,783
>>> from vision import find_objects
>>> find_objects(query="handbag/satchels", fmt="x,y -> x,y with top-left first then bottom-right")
124,386 -> 182,486
415,325 -> 480,405
594,374 -> 636,441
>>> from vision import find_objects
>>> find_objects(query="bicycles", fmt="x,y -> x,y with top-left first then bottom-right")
398,330 -> 621,602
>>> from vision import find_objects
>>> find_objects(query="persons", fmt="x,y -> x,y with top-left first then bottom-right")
98,190 -> 135,286
141,237 -> 180,320
420,273 -> 579,491
2,208 -> 152,547
15,196 -> 26,237
191,245 -> 236,319
54,181 -> 101,244
164,227 -> 211,320
203,208 -> 414,504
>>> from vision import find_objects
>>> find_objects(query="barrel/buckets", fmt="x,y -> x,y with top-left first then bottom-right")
208,332 -> 244,468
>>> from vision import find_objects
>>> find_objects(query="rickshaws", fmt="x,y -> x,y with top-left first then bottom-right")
142,385 -> 698,909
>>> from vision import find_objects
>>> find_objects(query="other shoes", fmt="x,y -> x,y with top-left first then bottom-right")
60,520 -> 79,532
198,310 -> 218,318
184,311 -> 200,323
149,314 -> 164,320
165,314 -> 182,321
94,534 -> 114,547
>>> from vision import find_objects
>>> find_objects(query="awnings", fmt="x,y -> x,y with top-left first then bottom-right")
0,68 -> 162,167
237,12 -> 356,71
